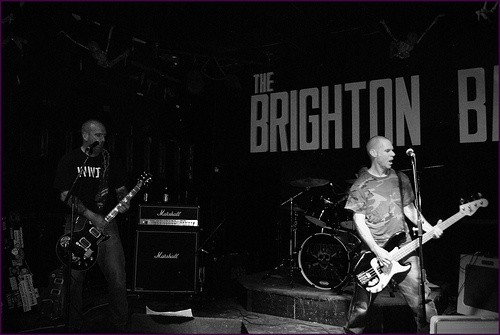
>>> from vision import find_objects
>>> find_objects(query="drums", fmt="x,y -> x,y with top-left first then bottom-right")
303,196 -> 336,230
333,197 -> 357,230
297,228 -> 362,291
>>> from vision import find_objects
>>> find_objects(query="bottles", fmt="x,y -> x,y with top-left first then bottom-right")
162,184 -> 169,206
143,183 -> 150,204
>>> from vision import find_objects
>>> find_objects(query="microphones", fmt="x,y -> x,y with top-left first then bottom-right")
302,186 -> 311,193
406,148 -> 416,157
86,141 -> 99,150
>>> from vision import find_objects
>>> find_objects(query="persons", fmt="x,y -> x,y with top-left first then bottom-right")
344,136 -> 443,335
56,120 -> 131,334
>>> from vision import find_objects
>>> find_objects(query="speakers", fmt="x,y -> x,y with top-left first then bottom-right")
126,312 -> 250,335
133,230 -> 199,293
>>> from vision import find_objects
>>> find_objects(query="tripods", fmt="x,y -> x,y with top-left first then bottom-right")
272,192 -> 305,288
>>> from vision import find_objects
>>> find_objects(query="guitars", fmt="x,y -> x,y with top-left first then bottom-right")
55,170 -> 153,272
353,192 -> 490,294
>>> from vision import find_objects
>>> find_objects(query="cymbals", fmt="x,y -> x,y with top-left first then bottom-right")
279,205 -> 307,212
289,178 -> 330,187
